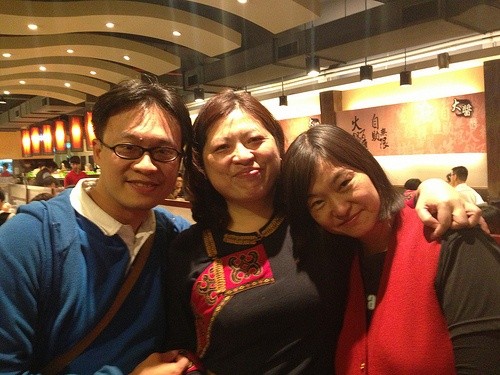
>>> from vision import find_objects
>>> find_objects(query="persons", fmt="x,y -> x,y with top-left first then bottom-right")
0,156 -> 193,225
402,166 -> 499,245
280,124 -> 500,375
166,90 -> 490,375
0,79 -> 192,375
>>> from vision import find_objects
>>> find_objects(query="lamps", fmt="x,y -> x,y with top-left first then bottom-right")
21,129 -> 31,157
438,53 -> 449,69
305,20 -> 320,76
279,75 -> 287,106
69,115 -> 83,152
30,126 -> 42,155
53,119 -> 67,154
42,123 -> 53,154
84,111 -> 97,151
194,84 -> 204,101
400,48 -> 412,86
360,0 -> 372,81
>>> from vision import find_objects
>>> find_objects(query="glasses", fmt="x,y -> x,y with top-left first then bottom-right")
98,139 -> 186,162
449,173 -> 456,178
